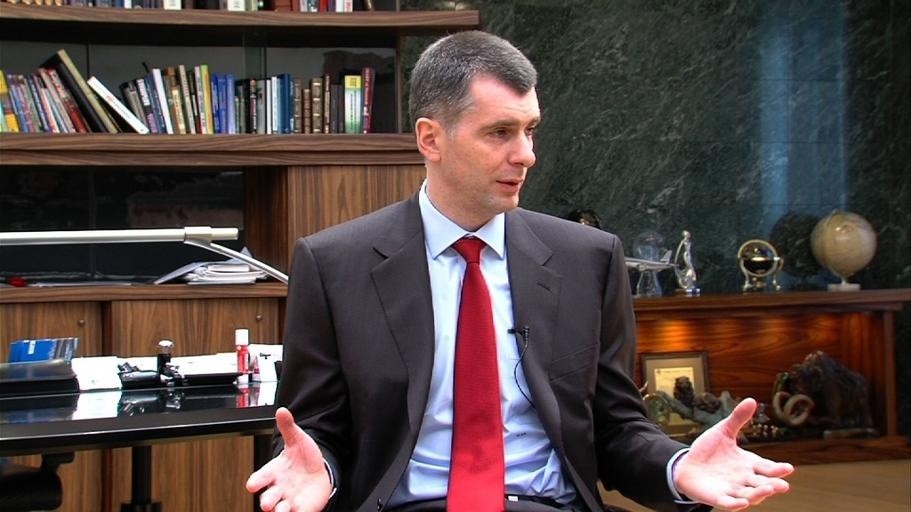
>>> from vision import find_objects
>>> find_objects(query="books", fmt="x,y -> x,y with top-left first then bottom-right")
178,258 -> 269,285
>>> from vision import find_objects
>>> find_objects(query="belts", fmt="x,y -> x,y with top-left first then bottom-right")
506,495 -> 566,510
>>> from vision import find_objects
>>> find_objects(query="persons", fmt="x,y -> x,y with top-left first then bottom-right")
245,32 -> 795,512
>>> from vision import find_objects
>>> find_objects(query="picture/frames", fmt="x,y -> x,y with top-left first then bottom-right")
640,351 -> 711,439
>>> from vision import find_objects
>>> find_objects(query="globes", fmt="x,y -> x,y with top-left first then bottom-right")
811,209 -> 877,291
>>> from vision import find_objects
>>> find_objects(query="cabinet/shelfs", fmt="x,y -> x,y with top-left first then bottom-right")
0,0 -> 482,511
625,285 -> 911,468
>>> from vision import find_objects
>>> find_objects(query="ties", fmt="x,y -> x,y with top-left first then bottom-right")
445,238 -> 505,512
